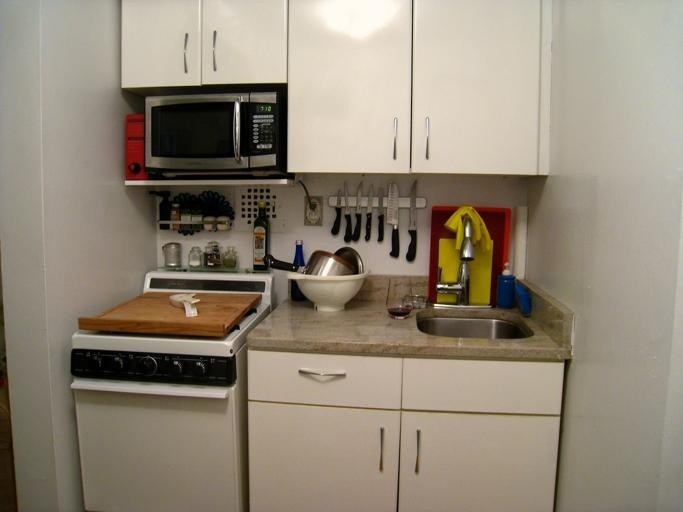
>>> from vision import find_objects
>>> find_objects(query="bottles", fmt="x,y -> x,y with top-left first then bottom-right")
290,240 -> 307,301
253,200 -> 270,271
203,216 -> 216,231
188,247 -> 202,267
217,216 -> 231,231
162,242 -> 183,268
223,246 -> 237,267
169,203 -> 181,230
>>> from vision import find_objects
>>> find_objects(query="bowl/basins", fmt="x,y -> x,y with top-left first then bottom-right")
286,271 -> 367,312
335,247 -> 363,274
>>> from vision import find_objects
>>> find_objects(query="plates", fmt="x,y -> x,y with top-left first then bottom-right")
169,292 -> 200,317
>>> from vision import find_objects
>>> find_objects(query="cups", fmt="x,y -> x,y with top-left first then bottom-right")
386,275 -> 413,320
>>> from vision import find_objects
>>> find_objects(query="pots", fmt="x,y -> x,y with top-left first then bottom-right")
263,250 -> 359,277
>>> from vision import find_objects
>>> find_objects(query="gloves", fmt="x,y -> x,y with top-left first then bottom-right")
455,210 -> 483,253
444,205 -> 491,252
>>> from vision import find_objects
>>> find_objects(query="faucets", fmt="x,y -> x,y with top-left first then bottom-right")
434,212 -> 474,306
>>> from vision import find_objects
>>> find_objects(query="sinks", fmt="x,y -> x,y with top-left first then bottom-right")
417,308 -> 535,339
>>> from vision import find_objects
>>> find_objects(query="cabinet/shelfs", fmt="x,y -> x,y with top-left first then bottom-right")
287,1 -> 549,175
120,0 -> 286,96
246,351 -> 564,512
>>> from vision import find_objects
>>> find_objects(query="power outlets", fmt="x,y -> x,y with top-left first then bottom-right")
303,196 -> 323,227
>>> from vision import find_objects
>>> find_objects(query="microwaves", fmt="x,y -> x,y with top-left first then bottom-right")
78,303 -> 265,342
145,92 -> 295,179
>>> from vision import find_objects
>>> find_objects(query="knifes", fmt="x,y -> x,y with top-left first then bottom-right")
378,184 -> 384,241
331,189 -> 342,235
353,180 -> 363,241
406,180 -> 419,262
387,182 -> 401,258
365,183 -> 374,241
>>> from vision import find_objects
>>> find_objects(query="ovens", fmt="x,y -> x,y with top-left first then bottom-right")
344,181 -> 352,242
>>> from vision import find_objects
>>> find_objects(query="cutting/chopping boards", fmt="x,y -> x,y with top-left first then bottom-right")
79,292 -> 263,340
